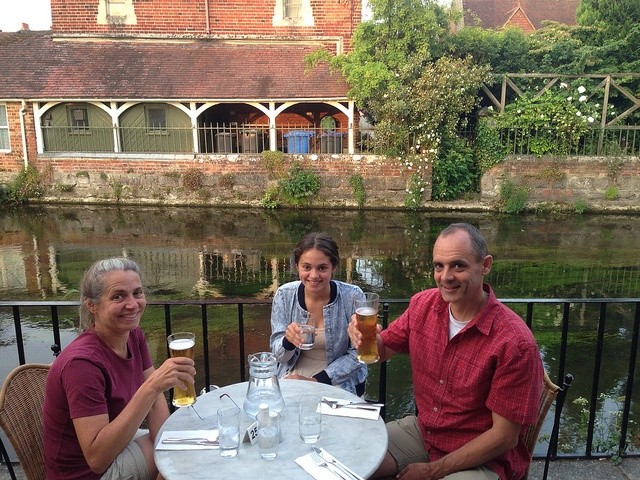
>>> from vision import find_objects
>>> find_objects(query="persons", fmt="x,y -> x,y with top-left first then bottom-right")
268,231 -> 368,398
345,222 -> 546,480
42,256 -> 196,480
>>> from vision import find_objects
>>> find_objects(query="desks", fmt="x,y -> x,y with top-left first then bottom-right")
152,379 -> 389,480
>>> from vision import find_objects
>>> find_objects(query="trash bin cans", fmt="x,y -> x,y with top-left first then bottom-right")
284,129 -> 314,154
239,130 -> 264,153
317,132 -> 346,153
215,133 -> 236,153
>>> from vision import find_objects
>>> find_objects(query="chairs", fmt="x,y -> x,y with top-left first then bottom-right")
1,344 -> 65,480
521,365 -> 573,479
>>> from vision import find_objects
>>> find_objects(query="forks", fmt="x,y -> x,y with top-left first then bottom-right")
322,398 -> 378,412
307,449 -> 347,480
164,436 -> 221,441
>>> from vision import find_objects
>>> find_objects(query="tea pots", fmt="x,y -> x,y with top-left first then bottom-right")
242,351 -> 285,421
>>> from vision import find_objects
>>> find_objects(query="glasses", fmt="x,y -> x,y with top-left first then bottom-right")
190,393 -> 240,424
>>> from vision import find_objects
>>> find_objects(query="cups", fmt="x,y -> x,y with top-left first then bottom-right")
217,406 -> 240,458
354,292 -> 380,365
167,331 -> 197,407
297,312 -> 315,350
299,394 -> 322,444
256,410 -> 281,462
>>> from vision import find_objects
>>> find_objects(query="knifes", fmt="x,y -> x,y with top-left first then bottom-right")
321,400 -> 385,407
161,439 -> 222,446
312,445 -> 362,480
